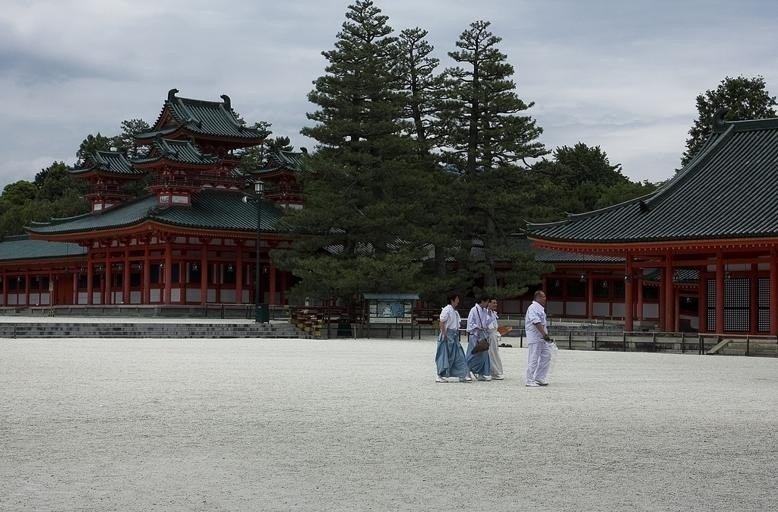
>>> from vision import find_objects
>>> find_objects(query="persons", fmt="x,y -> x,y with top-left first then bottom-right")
524,291 -> 553,387
465,296 -> 491,381
484,296 -> 504,381
434,293 -> 471,383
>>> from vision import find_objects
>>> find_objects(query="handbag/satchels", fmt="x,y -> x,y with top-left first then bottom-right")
470,338 -> 489,353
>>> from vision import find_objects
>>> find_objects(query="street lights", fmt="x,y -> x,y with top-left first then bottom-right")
241,179 -> 266,308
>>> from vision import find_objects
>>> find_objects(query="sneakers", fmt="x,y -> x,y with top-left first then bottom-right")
492,374 -> 503,380
459,376 -> 472,382
478,375 -> 490,380
436,377 -> 448,382
526,378 -> 548,387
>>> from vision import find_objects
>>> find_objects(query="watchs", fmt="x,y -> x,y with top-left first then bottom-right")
543,334 -> 548,338
